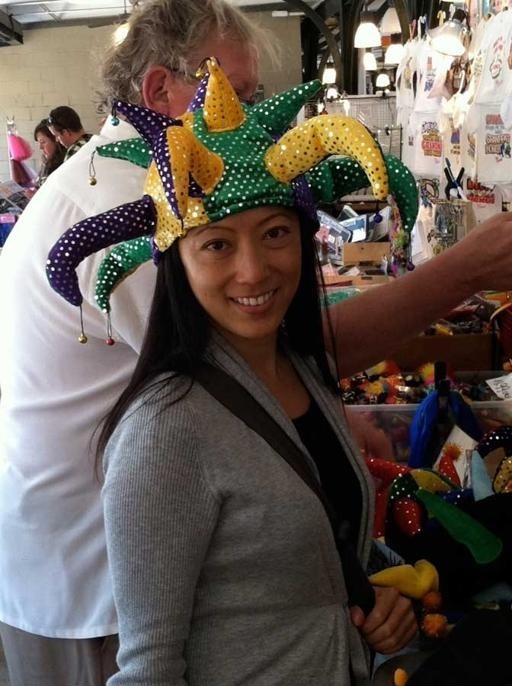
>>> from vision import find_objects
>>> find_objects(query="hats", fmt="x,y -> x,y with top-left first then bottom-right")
46,55 -> 418,344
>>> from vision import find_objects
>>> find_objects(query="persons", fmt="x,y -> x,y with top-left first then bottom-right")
0,0 -> 512,686
34,118 -> 66,187
46,106 -> 92,163
45,56 -> 418,686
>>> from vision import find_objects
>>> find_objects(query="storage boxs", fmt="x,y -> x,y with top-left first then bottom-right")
342,364 -> 511,465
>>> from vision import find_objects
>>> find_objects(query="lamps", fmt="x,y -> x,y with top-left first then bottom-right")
321,54 -> 337,99
353,0 -> 469,88
